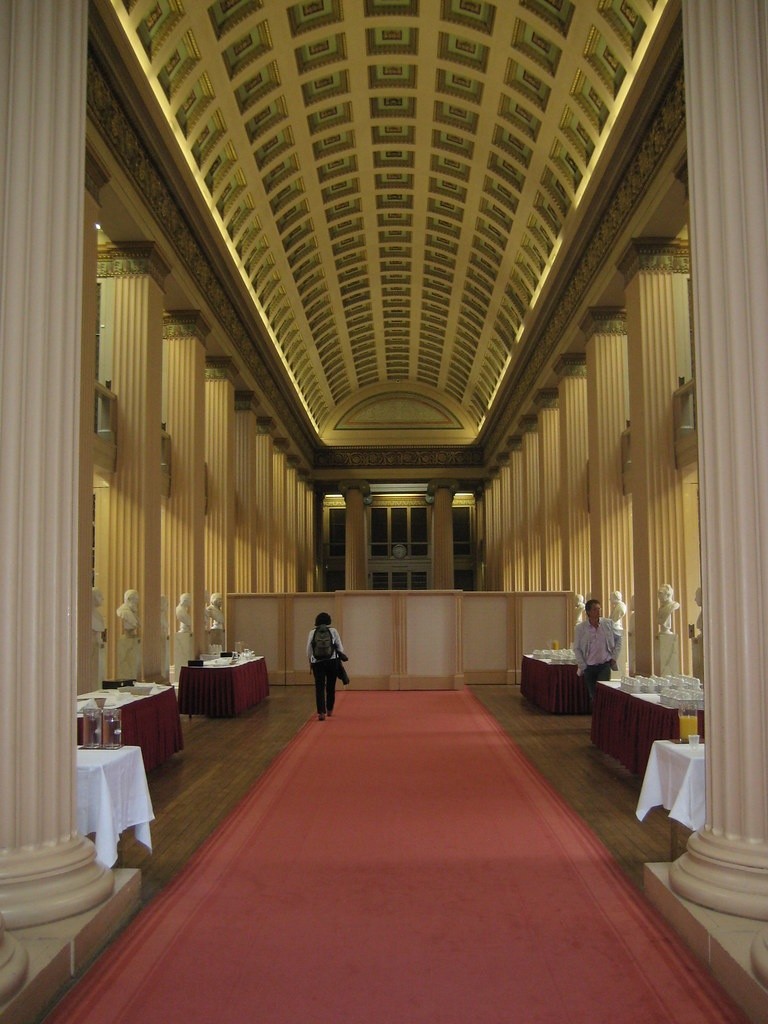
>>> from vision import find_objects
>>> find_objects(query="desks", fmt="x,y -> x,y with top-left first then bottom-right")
590,681 -> 704,780
635,739 -> 706,862
77,745 -> 155,868
76,682 -> 185,772
177,656 -> 269,719
520,655 -> 594,715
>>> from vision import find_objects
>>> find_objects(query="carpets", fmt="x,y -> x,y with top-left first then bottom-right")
33,685 -> 757,1023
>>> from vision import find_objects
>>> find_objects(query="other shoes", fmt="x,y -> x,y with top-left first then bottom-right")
319,714 -> 325,720
327,711 -> 332,716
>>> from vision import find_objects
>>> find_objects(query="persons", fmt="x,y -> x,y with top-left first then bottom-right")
206,592 -> 224,629
691,587 -> 704,648
628,595 -> 636,633
305,611 -> 350,720
176,593 -> 192,634
116,589 -> 140,630
656,583 -> 680,634
573,599 -> 622,714
609,590 -> 627,630
92,586 -> 105,633
160,595 -> 169,633
574,594 -> 585,626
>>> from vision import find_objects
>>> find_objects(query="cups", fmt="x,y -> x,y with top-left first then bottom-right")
94,698 -> 107,707
688,734 -> 700,753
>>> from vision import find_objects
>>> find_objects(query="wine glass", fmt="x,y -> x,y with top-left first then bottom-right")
232,652 -> 239,665
244,648 -> 250,662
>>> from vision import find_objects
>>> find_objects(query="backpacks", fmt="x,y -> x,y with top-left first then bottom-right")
312,624 -> 336,661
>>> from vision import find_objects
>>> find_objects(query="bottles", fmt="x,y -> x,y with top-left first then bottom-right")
252,651 -> 255,659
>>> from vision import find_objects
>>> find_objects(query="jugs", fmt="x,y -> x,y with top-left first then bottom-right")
103,708 -> 121,748
678,703 -> 697,742
82,708 -> 101,748
